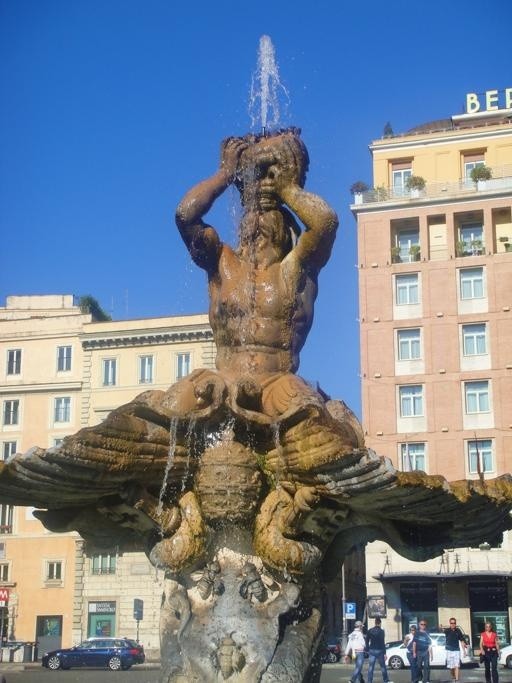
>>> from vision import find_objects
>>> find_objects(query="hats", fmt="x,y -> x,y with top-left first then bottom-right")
354,621 -> 363,627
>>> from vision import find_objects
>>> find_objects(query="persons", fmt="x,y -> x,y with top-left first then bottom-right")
480,621 -> 502,682
443,618 -> 468,683
132,136 -> 338,416
404,624 -> 422,682
344,621 -> 365,683
414,618 -> 433,682
365,617 -> 395,683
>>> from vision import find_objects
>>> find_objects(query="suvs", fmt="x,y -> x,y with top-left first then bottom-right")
40,637 -> 145,670
382,631 -> 476,668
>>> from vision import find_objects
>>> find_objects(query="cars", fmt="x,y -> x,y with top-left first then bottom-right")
496,644 -> 512,668
322,643 -> 340,664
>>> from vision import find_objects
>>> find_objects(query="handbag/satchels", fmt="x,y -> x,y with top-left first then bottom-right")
479,646 -> 487,663
352,654 -> 356,662
